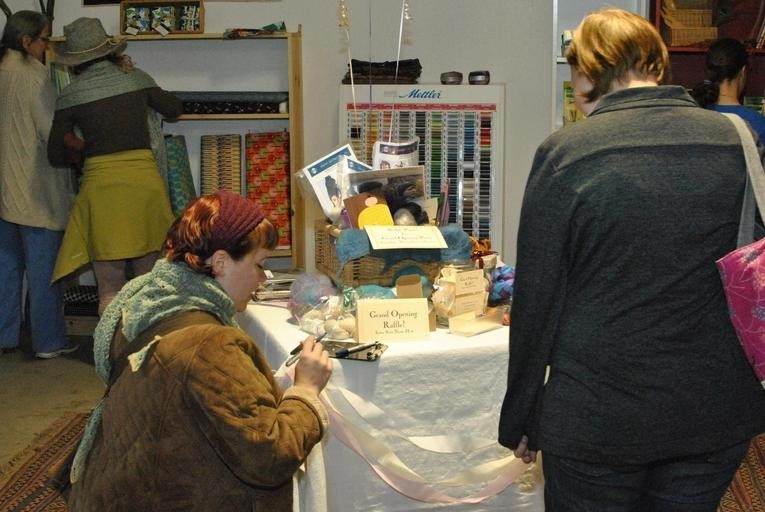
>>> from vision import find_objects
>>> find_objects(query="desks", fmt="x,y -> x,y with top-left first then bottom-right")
241,289 -> 546,508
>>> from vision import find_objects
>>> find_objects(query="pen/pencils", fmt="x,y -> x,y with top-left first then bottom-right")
286,332 -> 327,367
336,341 -> 378,356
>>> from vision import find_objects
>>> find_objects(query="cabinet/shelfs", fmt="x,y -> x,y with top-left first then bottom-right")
46,25 -> 306,276
549,1 -> 762,127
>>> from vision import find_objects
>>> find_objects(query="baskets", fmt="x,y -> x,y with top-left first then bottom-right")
315,216 -> 438,289
670,8 -> 718,47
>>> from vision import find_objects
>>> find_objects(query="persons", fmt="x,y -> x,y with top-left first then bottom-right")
50,18 -> 183,313
0,10 -> 79,360
47,193 -> 331,512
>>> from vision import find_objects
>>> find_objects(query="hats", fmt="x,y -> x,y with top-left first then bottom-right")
57,17 -> 129,66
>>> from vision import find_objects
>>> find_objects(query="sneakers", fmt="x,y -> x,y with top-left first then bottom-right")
35,343 -> 80,359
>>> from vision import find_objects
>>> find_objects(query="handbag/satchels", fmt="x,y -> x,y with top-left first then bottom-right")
715,237 -> 765,391
